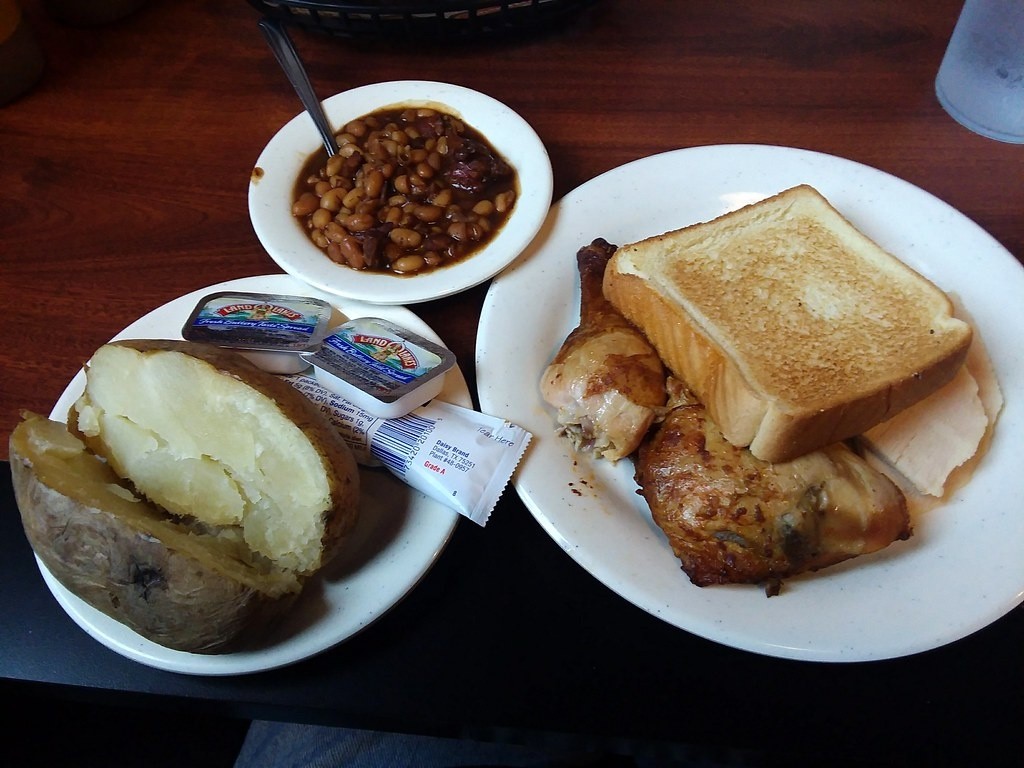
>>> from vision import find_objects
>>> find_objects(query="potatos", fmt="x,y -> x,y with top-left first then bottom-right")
7,410 -> 306,655
65,336 -> 360,578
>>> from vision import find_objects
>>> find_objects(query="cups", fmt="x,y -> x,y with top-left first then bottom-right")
935,0 -> 1024,145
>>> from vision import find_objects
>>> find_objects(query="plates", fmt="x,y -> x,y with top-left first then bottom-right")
13,269 -> 470,684
247,79 -> 553,306
474,142 -> 1023,660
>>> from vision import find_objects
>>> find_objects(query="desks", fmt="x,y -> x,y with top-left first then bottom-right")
0,0 -> 1024,766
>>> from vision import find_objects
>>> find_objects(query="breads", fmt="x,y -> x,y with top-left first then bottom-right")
599,185 -> 974,463
